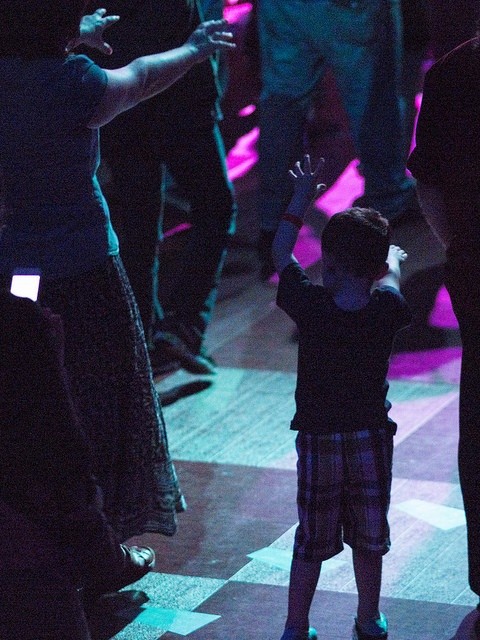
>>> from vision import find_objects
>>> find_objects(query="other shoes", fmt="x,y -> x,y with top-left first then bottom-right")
354,609 -> 389,638
81,545 -> 157,640
309,625 -> 318,639
153,320 -> 217,378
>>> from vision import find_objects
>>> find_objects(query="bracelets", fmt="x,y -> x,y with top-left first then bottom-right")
280,213 -> 304,230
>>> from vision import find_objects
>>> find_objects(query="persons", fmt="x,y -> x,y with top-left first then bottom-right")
0,0 -> 237,602
257,0 -> 419,260
406,38 -> 480,640
272,153 -> 415,640
0,207 -> 127,640
84,0 -> 237,379
